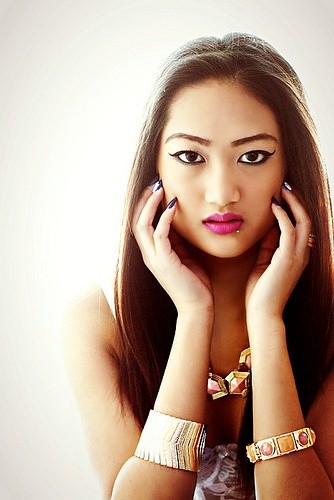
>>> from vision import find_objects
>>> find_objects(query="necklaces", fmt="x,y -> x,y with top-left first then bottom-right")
207,347 -> 250,400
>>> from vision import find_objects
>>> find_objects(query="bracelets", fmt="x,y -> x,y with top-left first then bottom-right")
246,428 -> 316,464
136,410 -> 206,474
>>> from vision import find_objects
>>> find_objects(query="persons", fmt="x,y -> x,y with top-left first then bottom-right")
76,34 -> 333,500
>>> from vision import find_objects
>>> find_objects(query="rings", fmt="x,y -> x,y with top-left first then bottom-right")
306,234 -> 315,250
